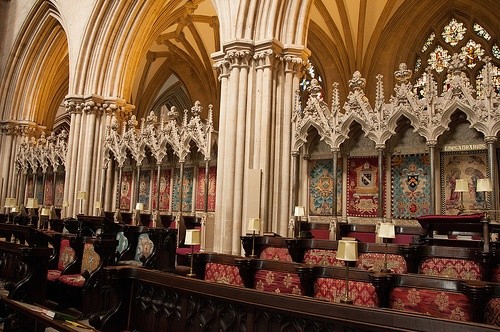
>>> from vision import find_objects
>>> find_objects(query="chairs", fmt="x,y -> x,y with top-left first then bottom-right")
0,213 -> 500,332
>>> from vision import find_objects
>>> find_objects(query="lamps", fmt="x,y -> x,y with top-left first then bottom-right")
184,229 -> 202,278
136,202 -> 143,214
476,178 -> 493,208
25,198 -> 38,226
336,239 -> 359,303
77,191 -> 87,215
294,206 -> 305,239
247,218 -> 261,258
4,198 -> 16,224
377,223 -> 396,273
454,179 -> 468,212
